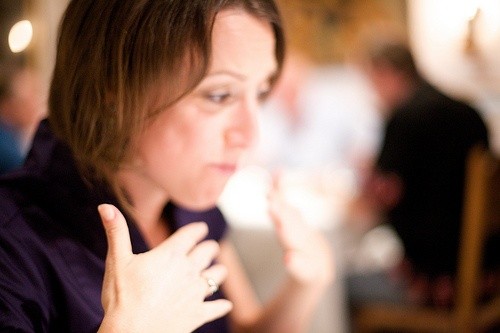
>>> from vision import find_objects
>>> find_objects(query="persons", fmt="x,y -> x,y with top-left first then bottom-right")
0,0 -> 500,333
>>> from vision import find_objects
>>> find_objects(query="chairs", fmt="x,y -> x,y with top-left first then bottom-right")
355,150 -> 500,333
